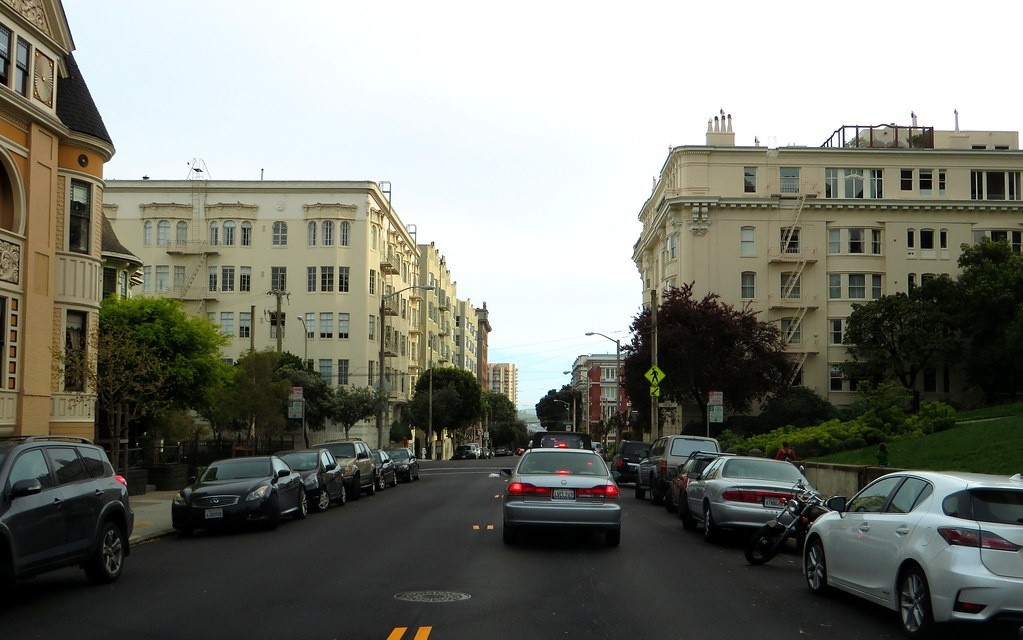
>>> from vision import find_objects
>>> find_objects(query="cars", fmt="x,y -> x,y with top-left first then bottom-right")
453,442 -> 525,460
273,448 -> 346,513
666,450 -> 738,520
372,449 -> 398,491
801,469 -> 1023,640
684,456 -> 820,546
382,448 -> 420,484
591,441 -> 606,461
611,440 -> 651,486
499,448 -> 622,547
171,454 -> 309,540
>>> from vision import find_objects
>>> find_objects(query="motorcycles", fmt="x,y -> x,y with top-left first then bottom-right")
744,465 -> 831,567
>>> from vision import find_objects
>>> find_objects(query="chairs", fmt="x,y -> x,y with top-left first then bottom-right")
568,440 -> 580,449
542,439 -> 553,448
725,470 -> 739,476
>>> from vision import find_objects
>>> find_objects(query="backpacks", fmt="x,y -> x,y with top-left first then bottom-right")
781,448 -> 792,462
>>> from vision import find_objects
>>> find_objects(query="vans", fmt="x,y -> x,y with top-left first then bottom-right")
531,431 -> 594,451
635,435 -> 722,505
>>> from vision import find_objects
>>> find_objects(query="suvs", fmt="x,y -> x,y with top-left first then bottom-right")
310,438 -> 377,501
0,435 -> 135,614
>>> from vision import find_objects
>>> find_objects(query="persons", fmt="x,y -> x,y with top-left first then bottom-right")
403,436 -> 408,448
777,442 -> 797,462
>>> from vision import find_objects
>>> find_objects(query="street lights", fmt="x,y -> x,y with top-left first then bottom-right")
554,399 -> 570,421
377,285 -> 436,449
562,370 -> 589,436
297,315 -> 309,371
583,332 -> 620,455
